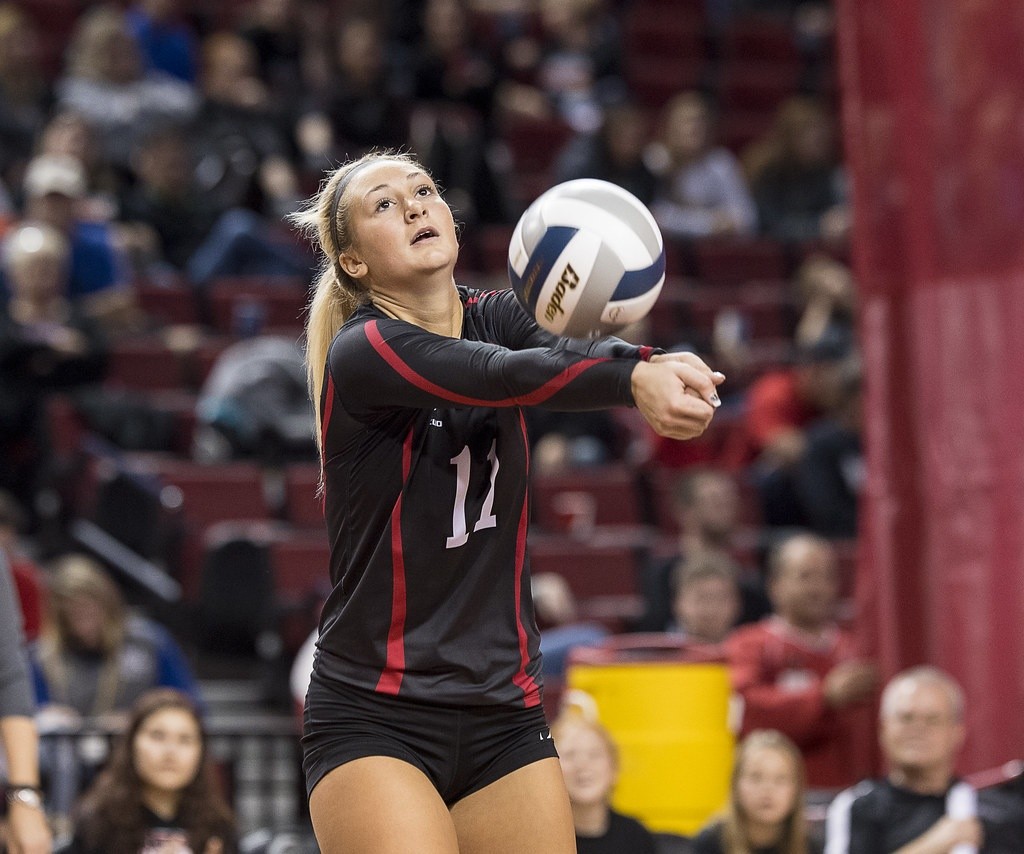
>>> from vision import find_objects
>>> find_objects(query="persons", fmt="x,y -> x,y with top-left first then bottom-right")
0,0 -> 1023,854
289,152 -> 725,854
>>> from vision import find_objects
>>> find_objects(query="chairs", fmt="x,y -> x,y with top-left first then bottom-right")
49,269 -> 879,652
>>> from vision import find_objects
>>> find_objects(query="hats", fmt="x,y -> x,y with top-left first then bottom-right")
23,152 -> 87,200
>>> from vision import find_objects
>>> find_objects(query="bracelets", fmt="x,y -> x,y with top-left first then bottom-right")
4,783 -> 43,808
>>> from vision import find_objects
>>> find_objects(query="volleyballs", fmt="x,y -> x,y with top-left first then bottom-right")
508,177 -> 665,342
